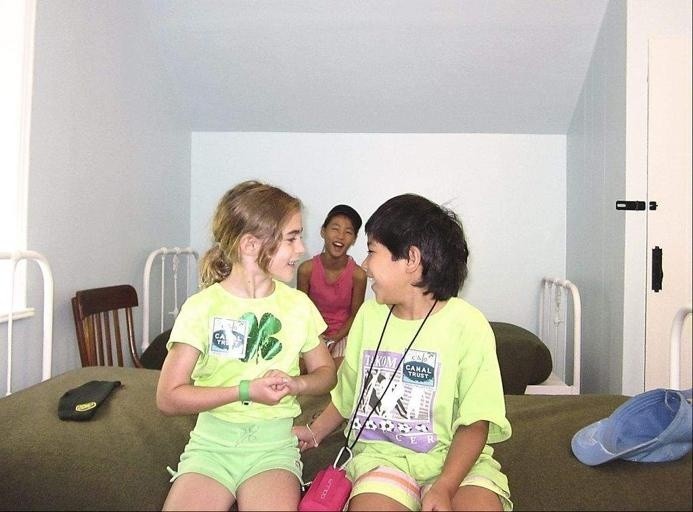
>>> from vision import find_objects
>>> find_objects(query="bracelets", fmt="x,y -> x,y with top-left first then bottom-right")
307,424 -> 319,447
239,380 -> 250,405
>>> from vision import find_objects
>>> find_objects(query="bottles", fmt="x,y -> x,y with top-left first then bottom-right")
324,205 -> 362,231
571,388 -> 692,467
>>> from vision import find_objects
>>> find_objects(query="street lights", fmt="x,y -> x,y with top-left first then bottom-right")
299,468 -> 352,512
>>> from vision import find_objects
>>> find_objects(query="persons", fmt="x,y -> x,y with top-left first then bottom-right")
157,182 -> 338,511
294,194 -> 511,512
297,205 -> 368,374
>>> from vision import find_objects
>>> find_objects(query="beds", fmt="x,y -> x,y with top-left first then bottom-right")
0,247 -> 691,512
